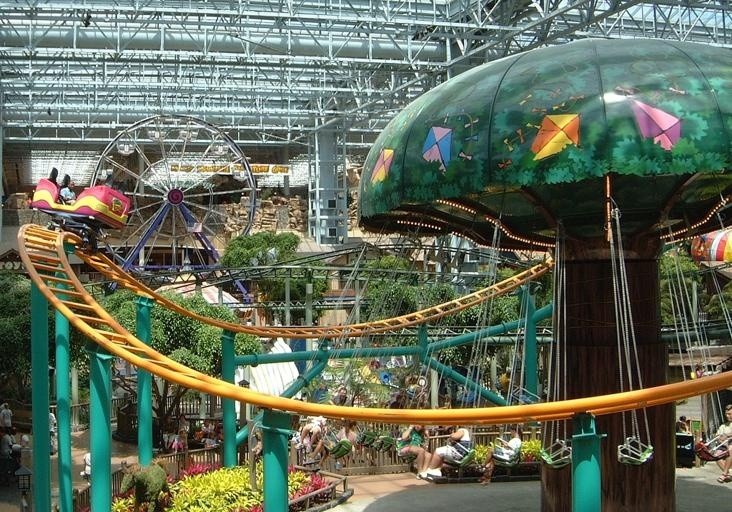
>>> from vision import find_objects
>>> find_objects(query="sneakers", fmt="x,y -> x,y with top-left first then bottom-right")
416,468 -> 442,481
295,443 -> 313,453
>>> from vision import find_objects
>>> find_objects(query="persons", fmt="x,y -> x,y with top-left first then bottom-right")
59,182 -> 76,205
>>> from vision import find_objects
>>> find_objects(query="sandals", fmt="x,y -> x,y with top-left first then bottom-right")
717,472 -> 732,483
